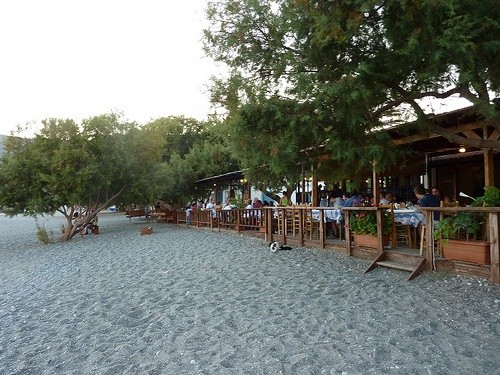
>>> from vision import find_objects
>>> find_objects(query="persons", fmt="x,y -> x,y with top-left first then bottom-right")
241,198 -> 275,225
185,203 -> 196,228
155,202 -> 160,213
380,192 -> 394,204
329,190 -> 369,240
413,185 -> 441,247
83,210 -> 98,235
280,191 -> 292,206
74,212 -> 83,238
199,198 -> 234,222
432,185 -> 455,206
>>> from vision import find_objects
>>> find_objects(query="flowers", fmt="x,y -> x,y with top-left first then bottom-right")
344,199 -> 392,234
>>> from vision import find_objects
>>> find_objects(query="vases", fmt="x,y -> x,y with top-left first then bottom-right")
354,233 -> 388,248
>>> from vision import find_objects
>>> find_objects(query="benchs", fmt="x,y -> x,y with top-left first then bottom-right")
126,210 -> 147,220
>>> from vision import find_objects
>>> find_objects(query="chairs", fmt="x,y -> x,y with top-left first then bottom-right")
277,205 -> 326,240
380,201 -> 444,256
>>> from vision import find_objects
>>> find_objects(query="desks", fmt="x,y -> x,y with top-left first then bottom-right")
308,209 -> 344,241
387,209 -> 425,227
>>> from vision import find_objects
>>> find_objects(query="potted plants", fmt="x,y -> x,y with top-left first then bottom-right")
434,186 -> 500,265
